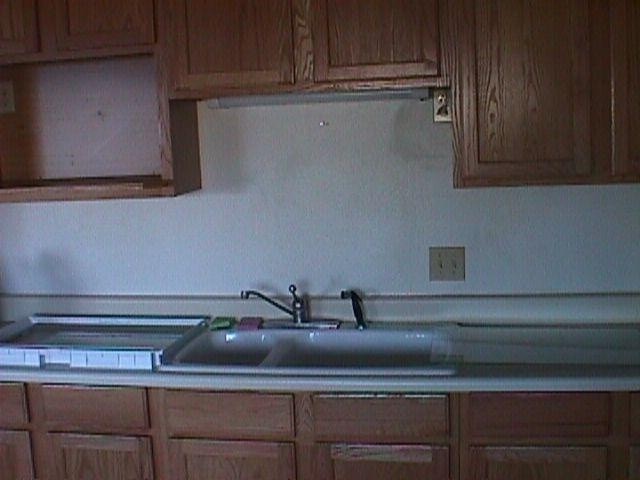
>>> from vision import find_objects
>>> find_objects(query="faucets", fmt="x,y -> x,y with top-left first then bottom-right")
240,284 -> 307,324
340,289 -> 369,330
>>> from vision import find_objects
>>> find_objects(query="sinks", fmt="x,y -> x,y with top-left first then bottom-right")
158,321 -> 274,374
274,323 -> 461,376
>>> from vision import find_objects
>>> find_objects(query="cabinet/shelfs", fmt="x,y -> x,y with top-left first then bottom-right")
0,0 -> 201,202
0,382 -> 640,480
162,0 -> 452,100
451,0 -> 640,186
